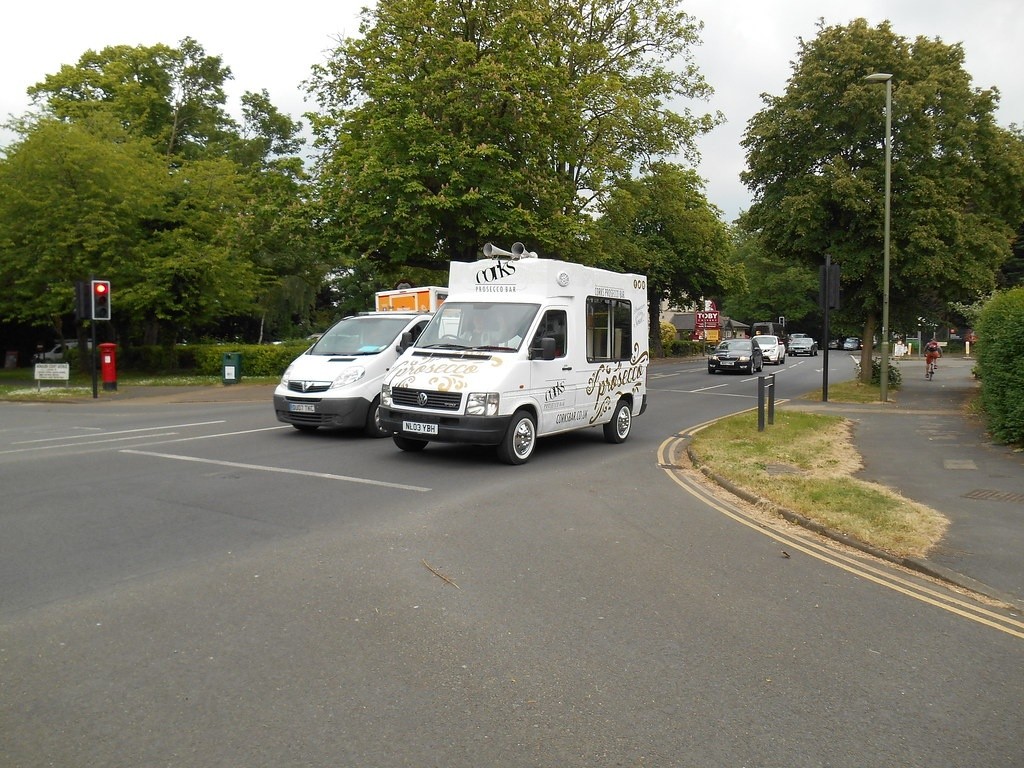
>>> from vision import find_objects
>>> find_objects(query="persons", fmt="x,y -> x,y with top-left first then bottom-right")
410,325 -> 422,342
924,339 -> 943,378
461,312 -> 505,346
756,331 -> 761,336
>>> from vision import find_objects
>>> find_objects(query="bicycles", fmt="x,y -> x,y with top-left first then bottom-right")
925,354 -> 941,381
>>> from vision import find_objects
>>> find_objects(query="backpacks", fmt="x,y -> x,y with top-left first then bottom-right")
928,342 -> 937,351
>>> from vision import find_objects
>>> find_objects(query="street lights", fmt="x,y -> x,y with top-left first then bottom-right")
865,73 -> 894,402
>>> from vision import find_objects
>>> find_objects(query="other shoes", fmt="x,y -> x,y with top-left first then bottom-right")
934,365 -> 938,368
925,374 -> 929,379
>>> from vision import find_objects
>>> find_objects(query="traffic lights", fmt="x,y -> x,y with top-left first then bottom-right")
90,280 -> 111,321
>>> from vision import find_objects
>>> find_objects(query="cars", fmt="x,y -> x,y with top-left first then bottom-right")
828,339 -> 843,349
787,338 -> 818,357
789,334 -> 809,347
751,335 -> 786,365
843,337 -> 861,350
707,338 -> 764,375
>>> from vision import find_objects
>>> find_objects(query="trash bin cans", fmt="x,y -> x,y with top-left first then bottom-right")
222,351 -> 242,386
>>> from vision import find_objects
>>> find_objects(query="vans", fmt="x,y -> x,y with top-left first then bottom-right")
272,310 -> 436,437
379,243 -> 649,465
752,321 -> 788,350
